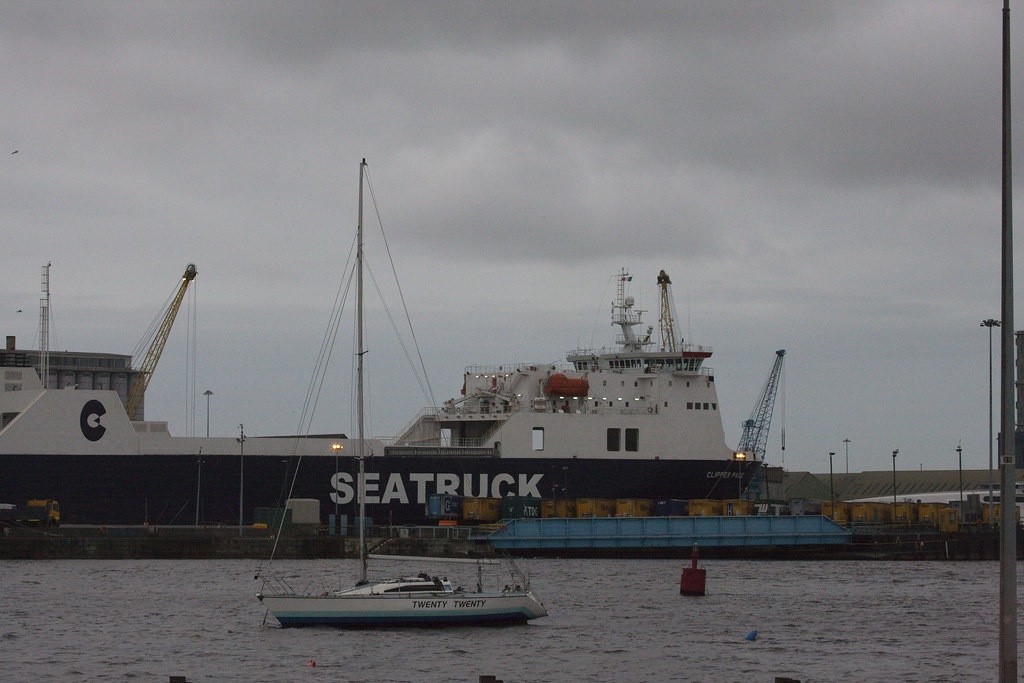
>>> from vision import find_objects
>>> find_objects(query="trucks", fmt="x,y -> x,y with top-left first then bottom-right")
15,498 -> 61,527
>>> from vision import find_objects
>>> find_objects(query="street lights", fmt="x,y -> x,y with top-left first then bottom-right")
892,448 -> 900,524
332,443 -> 343,515
829,452 -> 837,521
735,453 -> 745,516
979,317 -> 1002,528
236,424 -> 248,538
282,460 -> 289,501
955,445 -> 965,529
762,463 -> 770,515
194,447 -> 206,525
841,438 -> 853,499
203,390 -> 214,436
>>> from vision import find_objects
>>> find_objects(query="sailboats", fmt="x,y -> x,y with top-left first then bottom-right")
246,155 -> 549,629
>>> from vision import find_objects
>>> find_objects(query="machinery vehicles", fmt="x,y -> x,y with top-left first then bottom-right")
732,349 -> 787,516
108,261 -> 199,437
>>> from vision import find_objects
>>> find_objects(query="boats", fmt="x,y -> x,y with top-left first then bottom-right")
542,372 -> 590,398
0,261 -> 788,525
